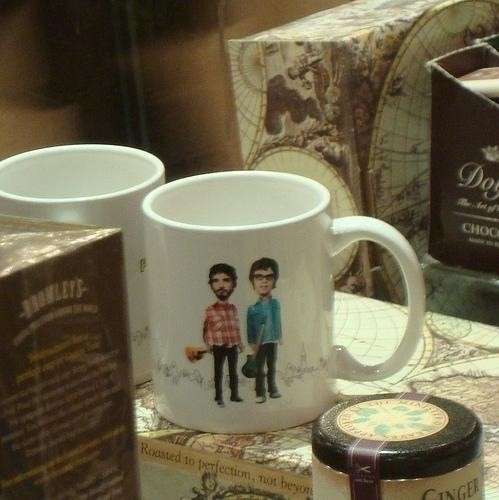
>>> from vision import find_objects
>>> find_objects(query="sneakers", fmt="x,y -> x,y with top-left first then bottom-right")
216,397 -> 243,407
271,393 -> 281,398
256,396 -> 266,403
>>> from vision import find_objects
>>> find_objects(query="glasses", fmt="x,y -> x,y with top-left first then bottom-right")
253,272 -> 275,281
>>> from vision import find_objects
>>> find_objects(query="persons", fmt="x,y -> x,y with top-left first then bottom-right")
202,264 -> 244,407
244,257 -> 286,406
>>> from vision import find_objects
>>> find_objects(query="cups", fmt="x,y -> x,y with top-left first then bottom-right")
142,170 -> 427,435
0,142 -> 166,390
